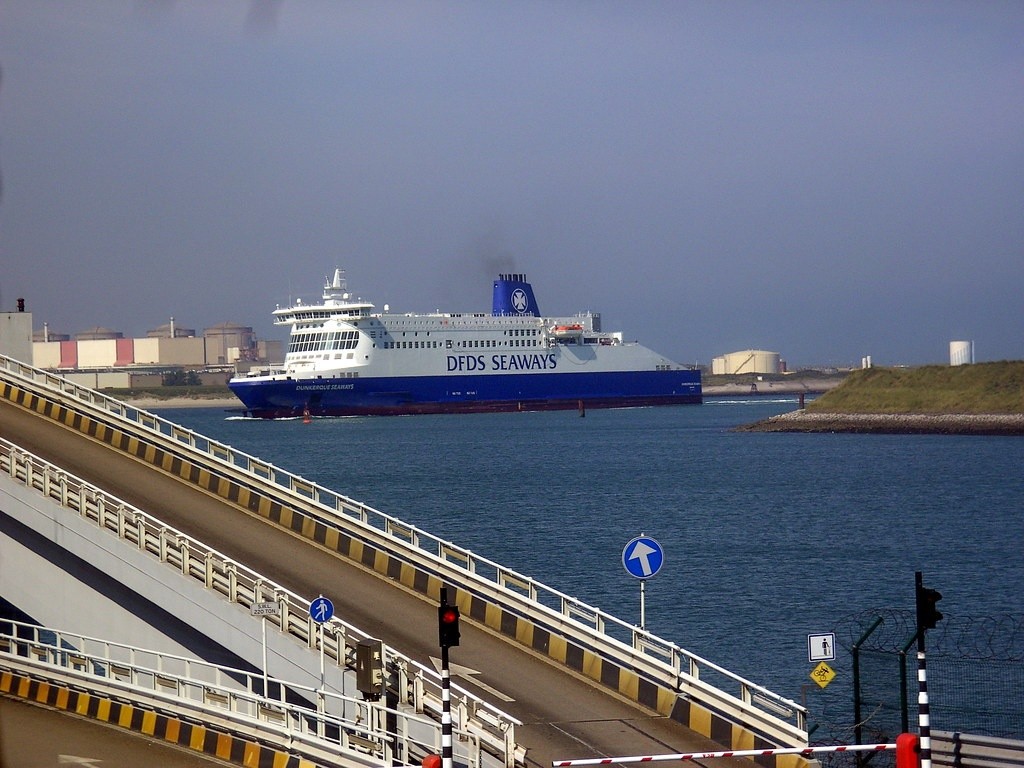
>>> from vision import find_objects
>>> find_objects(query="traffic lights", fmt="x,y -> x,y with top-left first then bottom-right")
921,587 -> 943,629
438,605 -> 460,647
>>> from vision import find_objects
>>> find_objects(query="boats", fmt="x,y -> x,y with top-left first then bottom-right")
549,325 -> 583,339
228,266 -> 706,419
